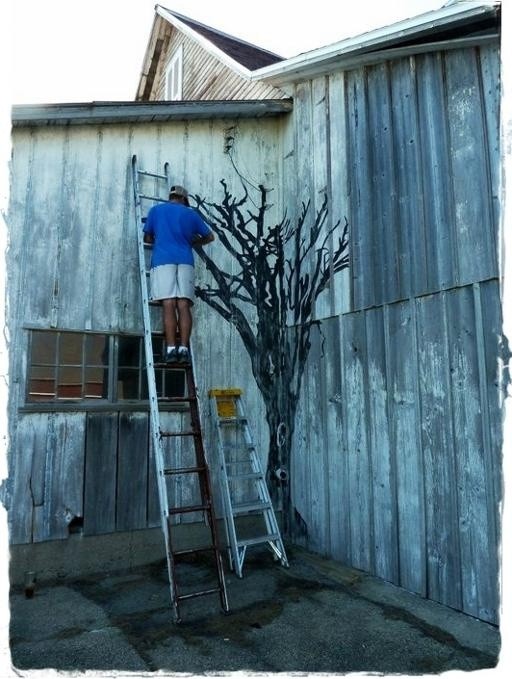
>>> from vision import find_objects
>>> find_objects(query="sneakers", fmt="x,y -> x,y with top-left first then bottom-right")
158,349 -> 190,364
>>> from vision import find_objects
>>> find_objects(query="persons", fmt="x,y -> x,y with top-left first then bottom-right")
143,185 -> 215,366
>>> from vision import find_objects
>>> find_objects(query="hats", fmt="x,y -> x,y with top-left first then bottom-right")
170,185 -> 191,207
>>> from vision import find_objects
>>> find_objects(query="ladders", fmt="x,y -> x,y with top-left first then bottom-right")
209,388 -> 290,579
132,154 -> 231,626
